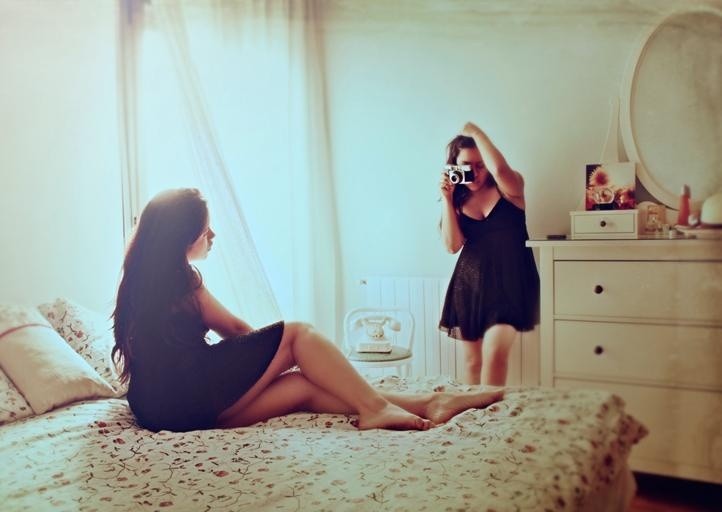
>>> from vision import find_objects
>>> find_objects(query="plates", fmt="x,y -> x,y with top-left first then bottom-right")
676,225 -> 721,240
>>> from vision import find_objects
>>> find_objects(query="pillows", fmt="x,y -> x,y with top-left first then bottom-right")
0,304 -> 117,415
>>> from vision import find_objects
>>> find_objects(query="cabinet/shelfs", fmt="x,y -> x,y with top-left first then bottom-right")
526,209 -> 722,484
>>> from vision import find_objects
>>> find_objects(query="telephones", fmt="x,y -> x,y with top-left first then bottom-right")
354,314 -> 400,353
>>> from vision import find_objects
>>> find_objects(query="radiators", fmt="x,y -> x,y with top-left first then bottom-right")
354,275 -> 539,387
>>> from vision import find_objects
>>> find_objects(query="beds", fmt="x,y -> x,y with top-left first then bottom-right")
0,377 -> 649,512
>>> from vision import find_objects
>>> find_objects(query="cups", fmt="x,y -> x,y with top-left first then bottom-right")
644,203 -> 667,233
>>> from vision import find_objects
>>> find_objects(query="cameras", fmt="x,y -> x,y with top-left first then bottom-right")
445,164 -> 474,184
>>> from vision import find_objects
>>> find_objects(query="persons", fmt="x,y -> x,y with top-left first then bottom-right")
107,187 -> 509,434
437,120 -> 540,384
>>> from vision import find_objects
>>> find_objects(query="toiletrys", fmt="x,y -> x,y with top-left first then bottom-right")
676,181 -> 690,224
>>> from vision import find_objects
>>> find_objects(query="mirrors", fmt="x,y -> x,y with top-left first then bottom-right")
617,0 -> 722,209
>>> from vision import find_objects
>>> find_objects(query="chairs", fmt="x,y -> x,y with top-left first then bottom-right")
344,306 -> 415,378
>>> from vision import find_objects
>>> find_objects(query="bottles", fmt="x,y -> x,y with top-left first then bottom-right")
676,185 -> 692,227
